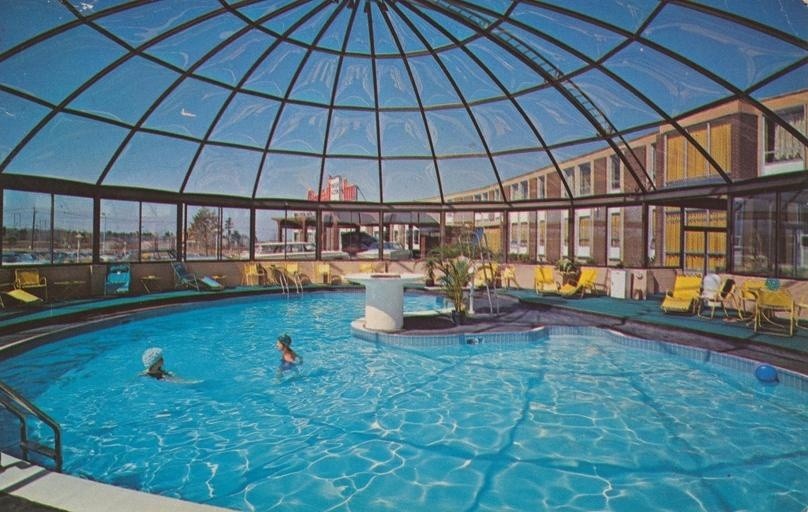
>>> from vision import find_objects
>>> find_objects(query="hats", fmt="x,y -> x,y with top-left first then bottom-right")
276,333 -> 292,348
142,347 -> 163,369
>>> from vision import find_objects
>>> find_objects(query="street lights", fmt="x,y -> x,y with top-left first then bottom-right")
284,200 -> 288,260
102,212 -> 106,255
74,231 -> 84,262
204,222 -> 208,257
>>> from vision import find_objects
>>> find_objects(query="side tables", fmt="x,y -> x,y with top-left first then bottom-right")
139,276 -> 162,295
53,281 -> 87,303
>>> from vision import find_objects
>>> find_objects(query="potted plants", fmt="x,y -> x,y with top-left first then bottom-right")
426,263 -> 434,287
412,246 -> 477,324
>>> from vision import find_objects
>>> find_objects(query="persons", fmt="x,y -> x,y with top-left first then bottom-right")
276,334 -> 301,374
138,347 -> 205,383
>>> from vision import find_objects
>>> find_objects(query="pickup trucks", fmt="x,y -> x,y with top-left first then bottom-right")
356,241 -> 413,262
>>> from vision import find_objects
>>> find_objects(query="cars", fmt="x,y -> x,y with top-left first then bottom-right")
255,242 -> 350,261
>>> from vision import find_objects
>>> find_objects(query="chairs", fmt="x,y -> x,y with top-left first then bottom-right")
103,262 -> 132,295
477,260 -> 519,290
534,267 -> 561,296
0,268 -> 49,310
659,271 -> 808,337
240,263 -> 342,292
171,261 -> 224,292
558,268 -> 598,299
